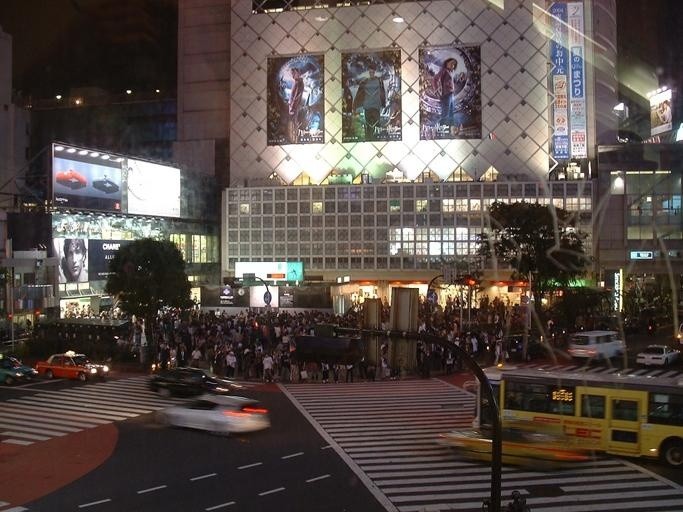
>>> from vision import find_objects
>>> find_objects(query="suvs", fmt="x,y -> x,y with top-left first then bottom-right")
566,328 -> 627,361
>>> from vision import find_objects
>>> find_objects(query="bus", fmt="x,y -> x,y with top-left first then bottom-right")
456,362 -> 682,471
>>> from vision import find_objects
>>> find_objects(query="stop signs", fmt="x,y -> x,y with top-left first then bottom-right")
7,313 -> 12,320
34,308 -> 41,316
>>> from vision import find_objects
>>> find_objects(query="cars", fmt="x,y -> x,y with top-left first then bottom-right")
33,348 -> 112,383
0,350 -> 39,387
636,344 -> 680,366
145,366 -> 243,400
677,324 -> 683,344
432,420 -> 603,476
153,393 -> 275,439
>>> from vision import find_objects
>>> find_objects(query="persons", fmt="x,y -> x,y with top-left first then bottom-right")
352,65 -> 387,141
656,100 -> 672,125
56,239 -> 88,282
432,58 -> 457,127
287,67 -> 304,144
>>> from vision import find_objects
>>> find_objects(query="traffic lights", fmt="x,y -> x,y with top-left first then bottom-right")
232,282 -> 242,288
463,276 -> 478,285
